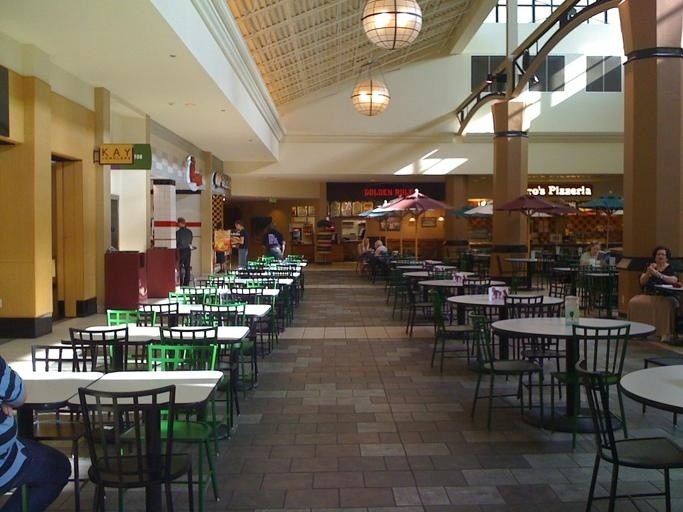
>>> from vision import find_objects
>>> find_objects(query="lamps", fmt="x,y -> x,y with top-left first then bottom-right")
351,43 -> 391,115
361,0 -> 424,50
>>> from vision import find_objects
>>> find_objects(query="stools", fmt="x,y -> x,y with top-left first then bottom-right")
319,251 -> 331,264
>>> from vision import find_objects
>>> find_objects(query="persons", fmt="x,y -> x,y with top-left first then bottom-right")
626,246 -> 679,344
0,356 -> 71,511
176,217 -> 193,286
216,228 -> 226,273
262,222 -> 287,260
366,238 -> 389,273
578,240 -> 606,309
231,220 -> 249,267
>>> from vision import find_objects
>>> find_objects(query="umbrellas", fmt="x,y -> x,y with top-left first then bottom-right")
356,187 -> 624,258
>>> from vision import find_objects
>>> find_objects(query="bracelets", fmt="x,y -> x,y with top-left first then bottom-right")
655,271 -> 663,279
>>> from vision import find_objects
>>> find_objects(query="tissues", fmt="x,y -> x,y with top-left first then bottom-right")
487,286 -> 508,301
